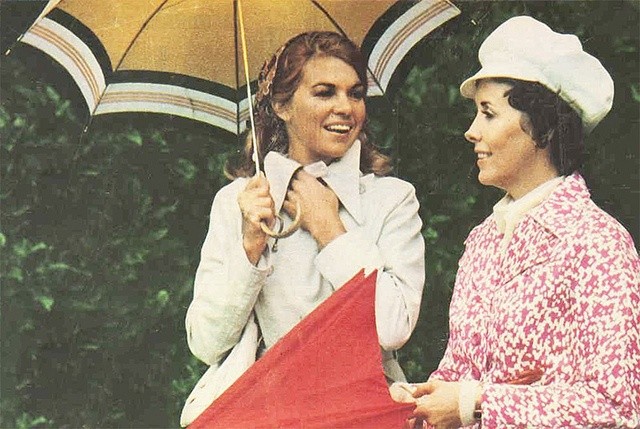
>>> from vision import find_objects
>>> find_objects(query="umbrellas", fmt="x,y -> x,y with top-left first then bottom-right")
181,267 -> 540,428
5,0 -> 464,239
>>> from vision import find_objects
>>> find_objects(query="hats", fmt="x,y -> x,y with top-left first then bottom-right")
459,15 -> 615,135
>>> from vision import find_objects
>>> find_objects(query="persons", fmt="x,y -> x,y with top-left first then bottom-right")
181,30 -> 427,426
390,15 -> 640,429
